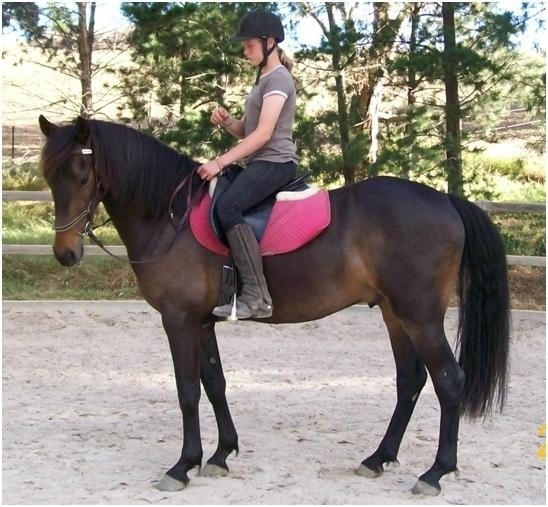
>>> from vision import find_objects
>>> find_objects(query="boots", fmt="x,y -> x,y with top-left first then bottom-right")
212,224 -> 274,319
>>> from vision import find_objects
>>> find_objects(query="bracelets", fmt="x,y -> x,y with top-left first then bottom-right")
213,156 -> 223,177
224,116 -> 232,126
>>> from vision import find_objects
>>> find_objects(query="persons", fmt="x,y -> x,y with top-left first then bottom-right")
195,7 -> 301,320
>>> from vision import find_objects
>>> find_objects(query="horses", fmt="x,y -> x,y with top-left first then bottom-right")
36,113 -> 515,497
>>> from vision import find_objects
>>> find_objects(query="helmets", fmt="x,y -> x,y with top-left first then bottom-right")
228,10 -> 284,43
